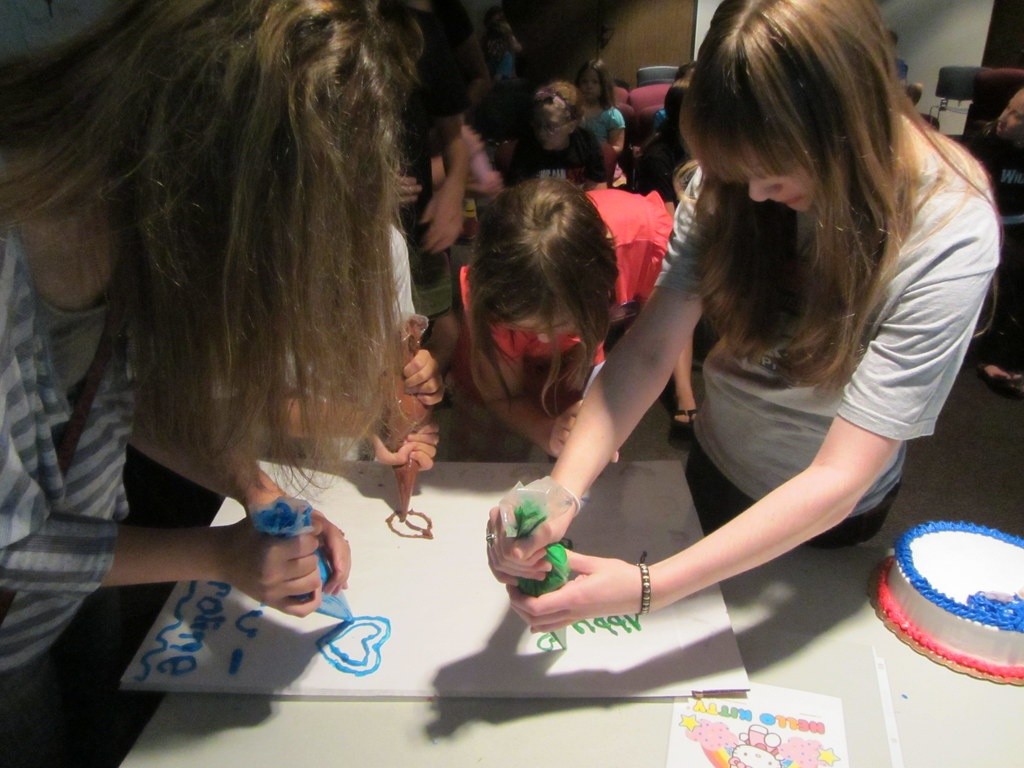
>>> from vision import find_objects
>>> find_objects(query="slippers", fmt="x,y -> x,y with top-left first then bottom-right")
673,408 -> 698,428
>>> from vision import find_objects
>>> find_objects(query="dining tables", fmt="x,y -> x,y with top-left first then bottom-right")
117,541 -> 1024,768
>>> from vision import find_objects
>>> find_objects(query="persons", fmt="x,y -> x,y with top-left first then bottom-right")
484,0 -> 1001,631
505,80 -> 606,191
575,58 -> 624,156
640,59 -> 698,437
128,214 -> 448,473
1,0 -> 412,768
975,88 -> 1024,387
458,179 -> 670,459
396,0 -> 524,370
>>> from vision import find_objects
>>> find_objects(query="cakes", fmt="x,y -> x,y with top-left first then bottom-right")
873,518 -> 1024,682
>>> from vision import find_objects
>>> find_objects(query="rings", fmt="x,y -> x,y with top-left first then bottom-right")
486,534 -> 494,541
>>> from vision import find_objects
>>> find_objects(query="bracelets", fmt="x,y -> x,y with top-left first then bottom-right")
638,550 -> 651,614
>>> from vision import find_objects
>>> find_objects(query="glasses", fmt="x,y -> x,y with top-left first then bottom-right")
535,118 -> 574,135
